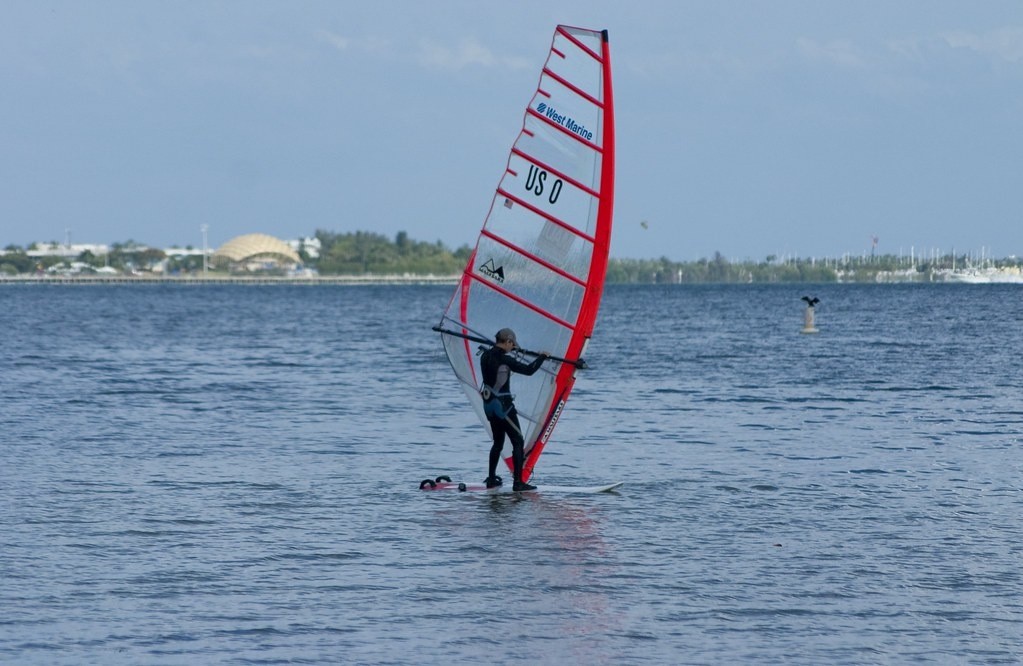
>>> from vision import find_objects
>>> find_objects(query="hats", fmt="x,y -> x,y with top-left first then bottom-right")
497,328 -> 519,347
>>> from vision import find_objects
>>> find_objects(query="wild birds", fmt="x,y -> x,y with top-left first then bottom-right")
800,296 -> 820,307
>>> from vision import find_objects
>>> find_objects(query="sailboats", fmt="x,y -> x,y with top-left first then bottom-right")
944,247 -> 998,283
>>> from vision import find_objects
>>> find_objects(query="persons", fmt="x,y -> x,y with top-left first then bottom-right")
480,328 -> 551,490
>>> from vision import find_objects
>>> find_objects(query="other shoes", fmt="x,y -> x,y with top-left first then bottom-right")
486,477 -> 503,488
513,482 -> 537,491
